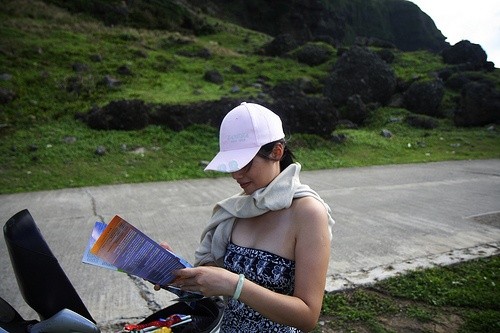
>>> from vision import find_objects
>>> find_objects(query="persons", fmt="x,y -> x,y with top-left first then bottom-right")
154,102 -> 335,333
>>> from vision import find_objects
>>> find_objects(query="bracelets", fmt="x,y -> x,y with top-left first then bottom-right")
232,274 -> 245,300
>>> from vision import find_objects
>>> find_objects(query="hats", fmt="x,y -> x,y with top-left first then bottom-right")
204,102 -> 285,173
